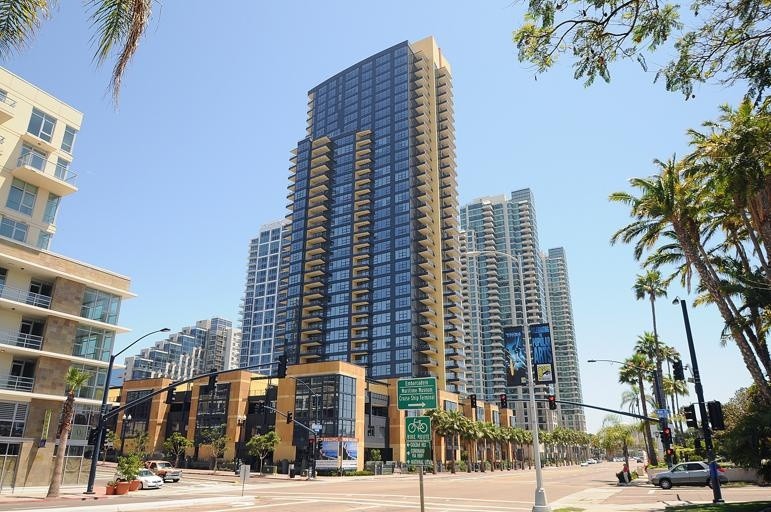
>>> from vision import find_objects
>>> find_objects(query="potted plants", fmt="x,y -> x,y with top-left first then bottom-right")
106,448 -> 146,495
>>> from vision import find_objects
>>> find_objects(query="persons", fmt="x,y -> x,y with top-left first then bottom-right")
623,463 -> 630,483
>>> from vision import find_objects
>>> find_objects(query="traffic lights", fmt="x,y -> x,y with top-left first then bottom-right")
471,393 -> 477,408
209,368 -> 218,390
663,427 -> 672,458
260,405 -> 264,415
684,404 -> 697,427
287,411 -> 293,423
500,394 -> 507,409
278,355 -> 288,378
673,360 -> 684,380
166,384 -> 176,404
549,395 -> 557,410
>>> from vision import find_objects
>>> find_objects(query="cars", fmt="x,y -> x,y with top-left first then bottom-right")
114,460 -> 180,489
652,461 -> 728,489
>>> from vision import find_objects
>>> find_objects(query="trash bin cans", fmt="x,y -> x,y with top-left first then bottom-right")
281,459 -> 289,474
185,455 -> 191,469
437,461 -> 442,472
235,459 -> 242,474
375,461 -> 384,475
475,463 -> 479,472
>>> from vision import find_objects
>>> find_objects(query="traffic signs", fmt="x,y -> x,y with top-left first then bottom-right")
397,377 -> 438,410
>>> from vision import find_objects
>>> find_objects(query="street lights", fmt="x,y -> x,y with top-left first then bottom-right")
466,251 -> 548,512
672,296 -> 723,501
235,414 -> 246,474
286,375 -> 318,478
120,413 -> 132,455
587,360 -> 673,467
87,327 -> 170,494
